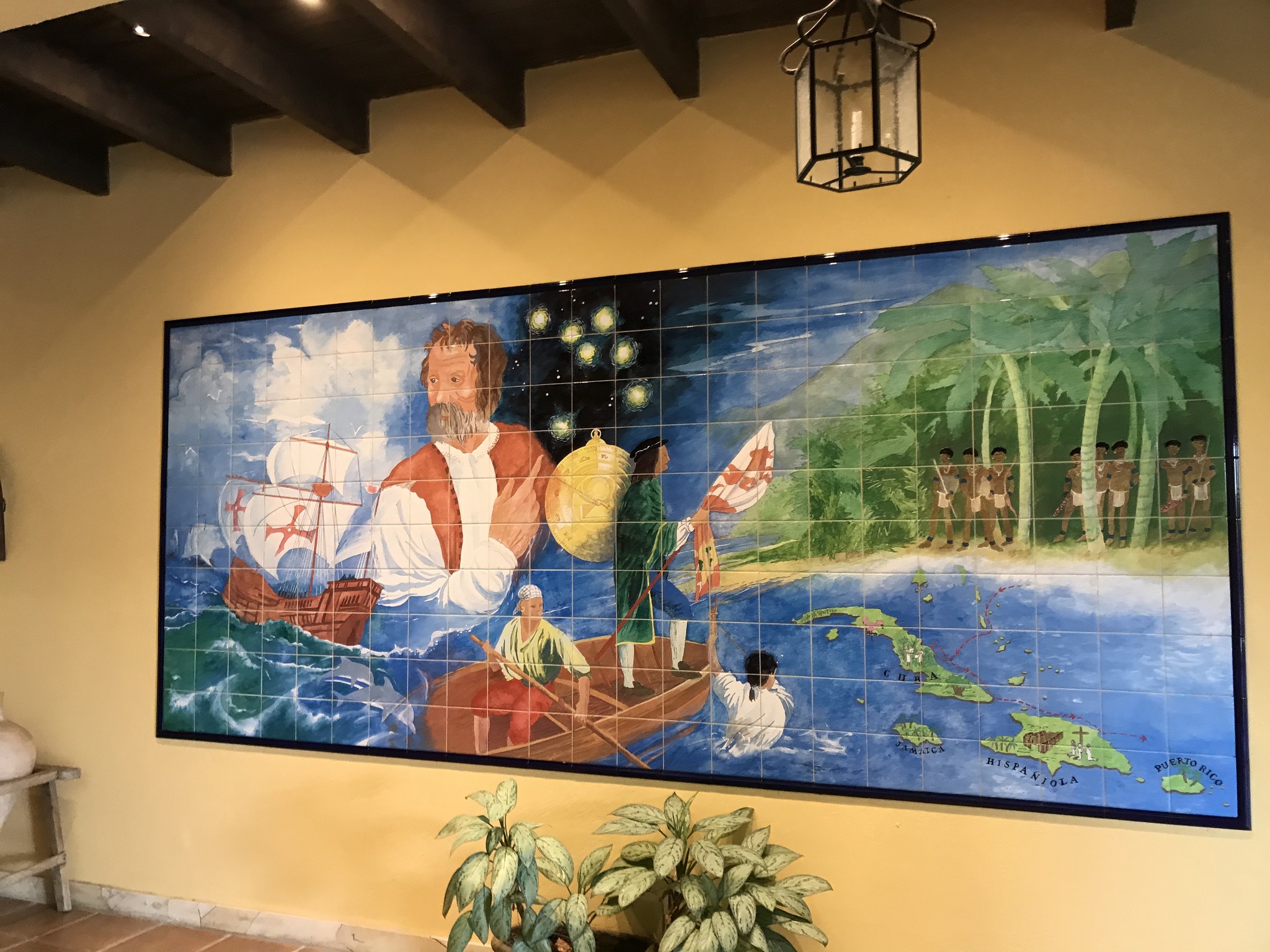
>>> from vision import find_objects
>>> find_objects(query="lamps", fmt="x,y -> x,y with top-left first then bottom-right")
777,0 -> 936,193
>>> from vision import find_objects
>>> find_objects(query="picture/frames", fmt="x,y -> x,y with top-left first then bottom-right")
157,209 -> 1252,830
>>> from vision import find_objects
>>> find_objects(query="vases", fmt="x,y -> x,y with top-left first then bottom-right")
1,689 -> 37,832
